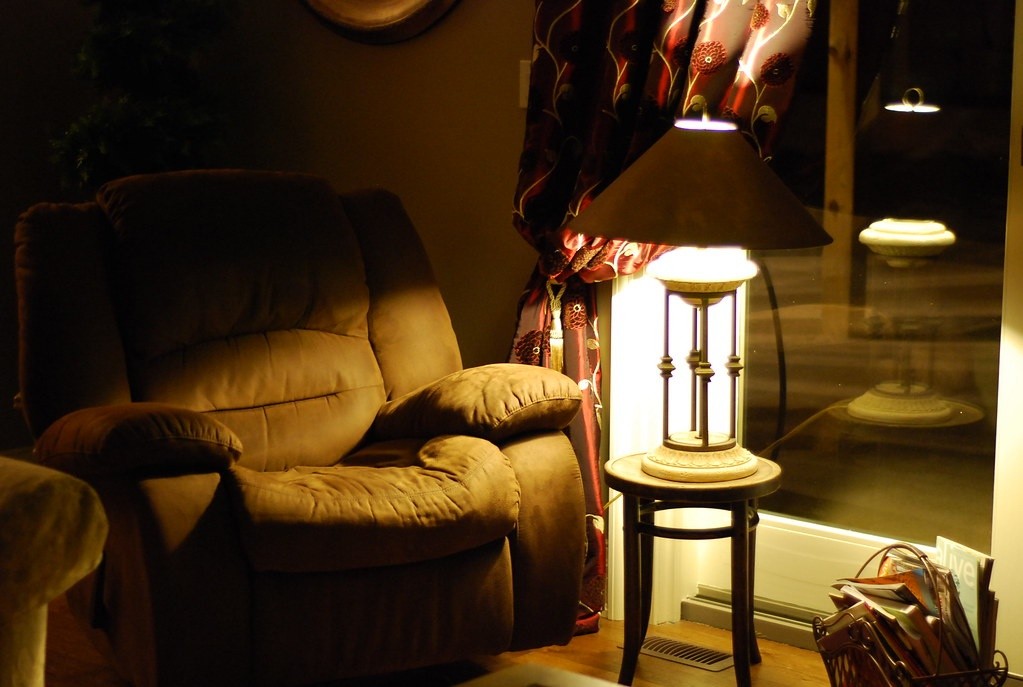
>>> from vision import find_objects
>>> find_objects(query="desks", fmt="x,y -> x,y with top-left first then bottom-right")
601,454 -> 784,687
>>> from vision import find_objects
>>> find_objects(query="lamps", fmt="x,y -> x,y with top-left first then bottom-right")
798,85 -> 1006,427
567,111 -> 834,478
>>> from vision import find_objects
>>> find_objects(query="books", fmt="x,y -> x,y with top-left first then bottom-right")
812,536 -> 999,687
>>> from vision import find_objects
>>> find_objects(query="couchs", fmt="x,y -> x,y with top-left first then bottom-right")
16,169 -> 587,687
